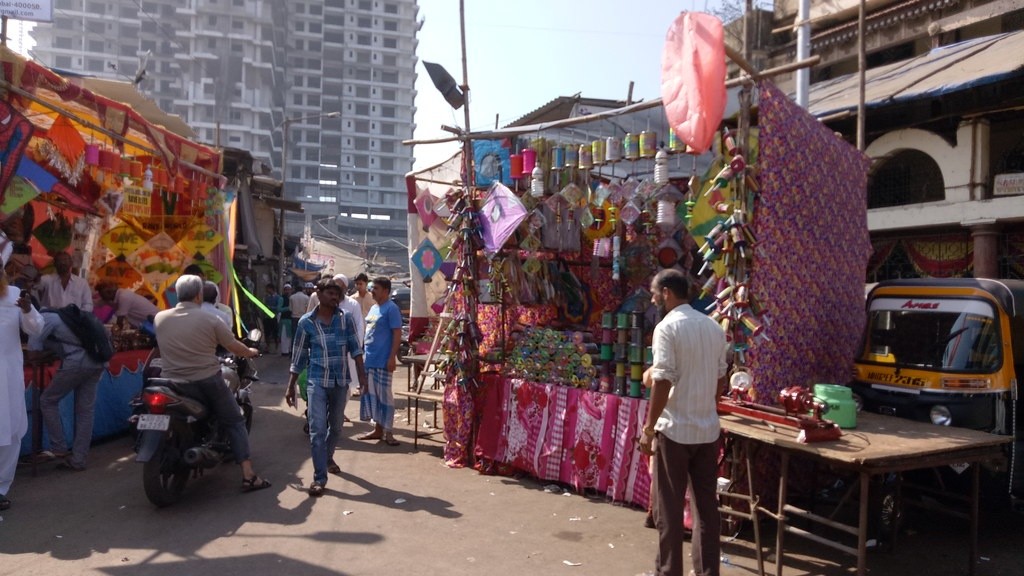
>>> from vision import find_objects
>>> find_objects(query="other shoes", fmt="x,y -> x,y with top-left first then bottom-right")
384,434 -> 400,446
309,481 -> 326,496
358,430 -> 385,439
54,462 -> 87,472
303,409 -> 310,435
327,459 -> 340,475
35,451 -> 67,459
0,492 -> 11,510
343,415 -> 350,422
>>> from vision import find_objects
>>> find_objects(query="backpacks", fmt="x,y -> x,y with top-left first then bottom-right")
39,301 -> 116,366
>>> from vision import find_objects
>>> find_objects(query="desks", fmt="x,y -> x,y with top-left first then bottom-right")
472,371 -> 778,535
402,354 -> 449,427
718,410 -> 1013,576
18,345 -> 158,456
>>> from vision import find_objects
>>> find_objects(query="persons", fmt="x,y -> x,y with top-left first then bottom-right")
639,270 -> 727,575
349,273 -> 376,396
185,265 -> 220,300
263,281 -> 313,356
34,252 -> 93,312
0,254 -> 44,511
356,277 -> 403,447
91,281 -> 159,331
285,278 -> 369,497
200,282 -> 230,326
299,274 -> 364,435
20,290 -> 98,471
154,274 -> 272,493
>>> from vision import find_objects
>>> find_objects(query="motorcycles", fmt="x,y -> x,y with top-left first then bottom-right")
844,277 -> 1023,518
125,328 -> 263,504
391,280 -> 415,364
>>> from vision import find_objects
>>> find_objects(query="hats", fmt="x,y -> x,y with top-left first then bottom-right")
315,279 -> 340,290
305,283 -> 314,288
333,274 -> 349,288
282,284 -> 293,289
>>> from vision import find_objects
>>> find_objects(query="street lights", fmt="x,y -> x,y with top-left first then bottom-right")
275,110 -> 343,347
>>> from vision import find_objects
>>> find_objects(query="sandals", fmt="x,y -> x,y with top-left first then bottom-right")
240,476 -> 271,491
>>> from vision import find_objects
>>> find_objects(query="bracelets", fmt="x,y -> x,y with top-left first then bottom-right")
642,426 -> 654,435
